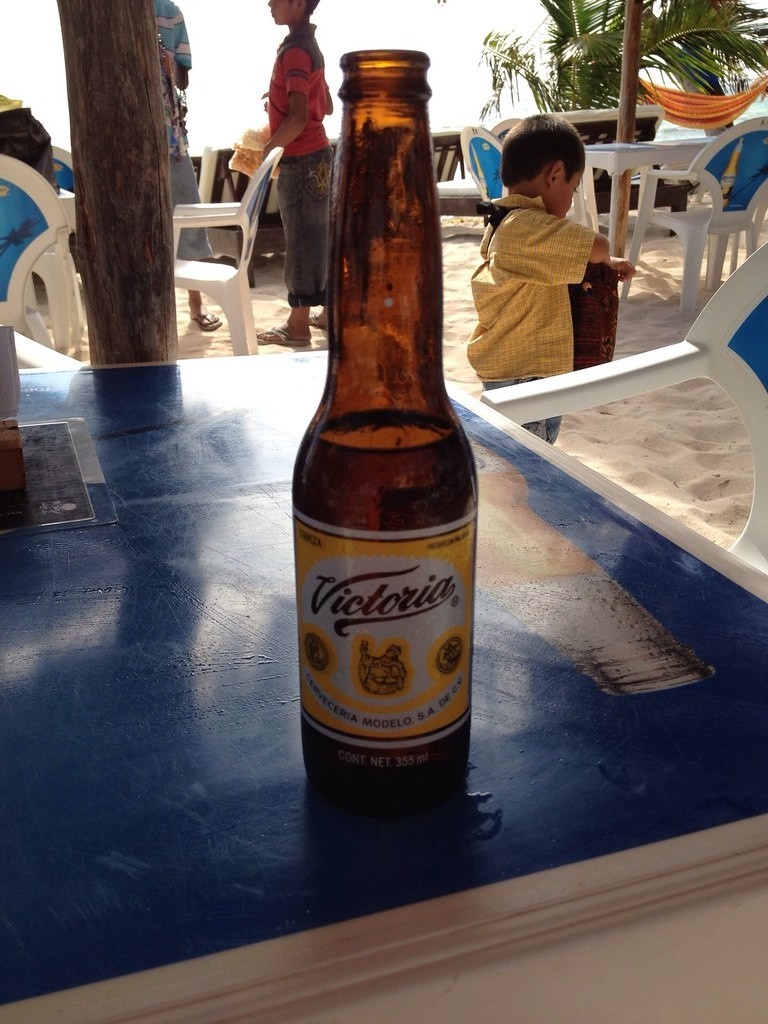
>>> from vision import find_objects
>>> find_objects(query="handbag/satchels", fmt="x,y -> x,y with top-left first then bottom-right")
567,263 -> 620,367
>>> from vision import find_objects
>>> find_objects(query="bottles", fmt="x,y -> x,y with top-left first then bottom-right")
292,49 -> 480,813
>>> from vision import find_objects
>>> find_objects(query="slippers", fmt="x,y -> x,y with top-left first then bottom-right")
194,311 -> 222,331
257,326 -> 311,347
310,316 -> 326,330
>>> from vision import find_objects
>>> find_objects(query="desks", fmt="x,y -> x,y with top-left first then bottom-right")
582,135 -> 715,266
0,348 -> 768,1024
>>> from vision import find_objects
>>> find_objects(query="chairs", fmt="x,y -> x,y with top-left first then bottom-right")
479,241 -> 768,573
461,118 -> 603,238
172,146 -> 284,354
0,146 -> 89,367
619,117 -> 768,314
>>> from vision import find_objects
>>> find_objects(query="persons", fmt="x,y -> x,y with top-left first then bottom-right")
466,115 -> 636,445
254,0 -> 336,348
151,0 -> 223,330
0,93 -> 61,195
